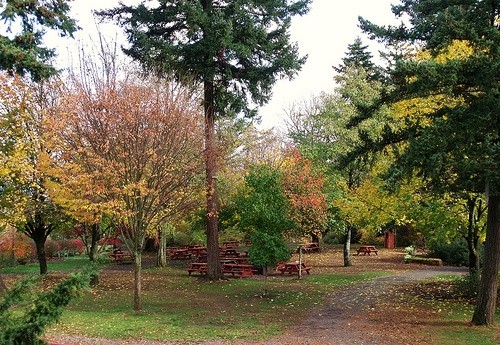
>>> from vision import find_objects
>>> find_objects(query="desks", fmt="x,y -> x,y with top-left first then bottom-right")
357,246 -> 378,256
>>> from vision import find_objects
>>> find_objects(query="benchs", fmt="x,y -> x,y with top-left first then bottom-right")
355,249 -> 366,252
367,250 -> 379,253
110,239 -> 319,279
